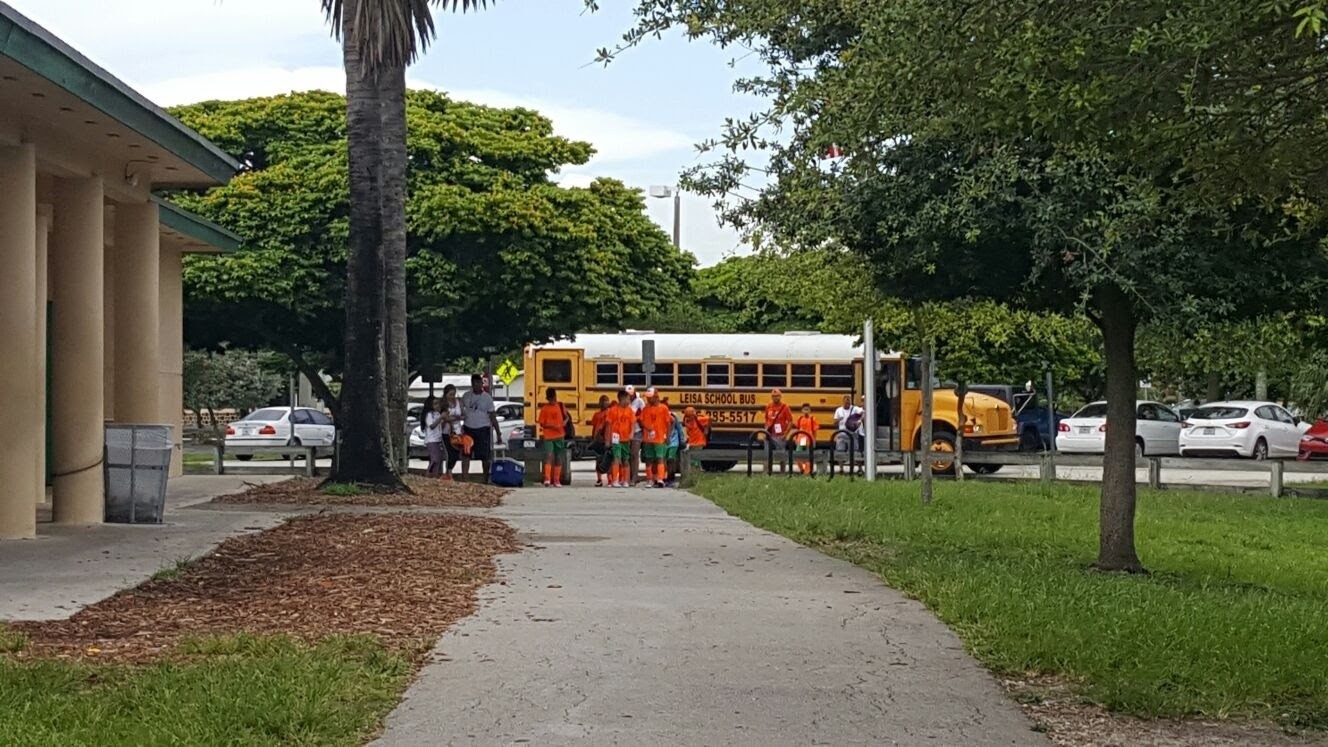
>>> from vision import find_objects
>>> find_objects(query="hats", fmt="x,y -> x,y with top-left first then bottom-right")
682,407 -> 696,416
645,388 -> 658,397
600,395 -> 609,401
625,385 -> 634,395
472,374 -> 483,379
771,388 -> 781,395
546,389 -> 555,399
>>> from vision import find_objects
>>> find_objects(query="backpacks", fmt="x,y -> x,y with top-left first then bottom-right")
560,403 -> 575,440
846,406 -> 861,431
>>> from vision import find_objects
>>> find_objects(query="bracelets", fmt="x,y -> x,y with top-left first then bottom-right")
498,433 -> 501,435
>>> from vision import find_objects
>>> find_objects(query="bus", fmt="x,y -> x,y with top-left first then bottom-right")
519,328 -> 1024,476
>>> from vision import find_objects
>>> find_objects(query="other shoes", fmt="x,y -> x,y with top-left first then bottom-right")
441,474 -> 452,480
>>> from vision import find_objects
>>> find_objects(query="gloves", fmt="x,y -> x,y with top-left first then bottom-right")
535,440 -> 543,448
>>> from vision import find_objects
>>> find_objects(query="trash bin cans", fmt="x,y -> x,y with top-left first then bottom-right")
105,423 -> 175,524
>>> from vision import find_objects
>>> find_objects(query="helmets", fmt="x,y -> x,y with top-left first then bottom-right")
449,433 -> 474,450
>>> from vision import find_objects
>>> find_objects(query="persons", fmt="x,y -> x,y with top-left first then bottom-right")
459,374 -> 503,484
791,403 -> 819,476
832,394 -> 865,453
590,384 -> 684,489
854,396 -> 865,452
420,395 -> 449,479
761,388 -> 793,475
440,384 -> 466,482
682,407 -> 709,472
538,387 -> 569,489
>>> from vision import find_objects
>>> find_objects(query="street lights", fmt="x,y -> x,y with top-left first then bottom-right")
648,183 -> 681,253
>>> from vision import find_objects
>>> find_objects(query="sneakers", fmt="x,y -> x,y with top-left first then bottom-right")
544,480 -> 550,487
608,480 -> 629,488
553,481 -> 561,487
645,477 -> 676,488
595,480 -> 602,486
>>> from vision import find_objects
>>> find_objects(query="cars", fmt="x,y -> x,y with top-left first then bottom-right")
1054,398 -> 1185,463
222,405 -> 337,461
1179,399 -> 1315,461
404,372 -> 524,465
1294,415 -> 1328,462
